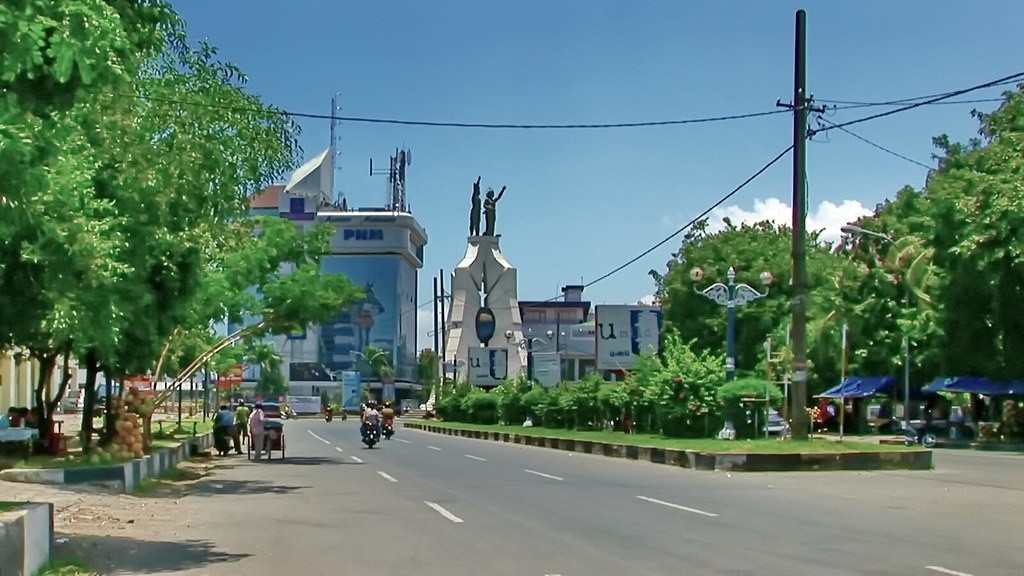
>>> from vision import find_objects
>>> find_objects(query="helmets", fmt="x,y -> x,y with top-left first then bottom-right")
343,405 -> 345,408
368,399 -> 376,407
286,404 -> 289,407
328,403 -> 330,406
385,402 -> 391,407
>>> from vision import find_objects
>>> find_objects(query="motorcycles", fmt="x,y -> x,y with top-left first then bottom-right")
383,418 -> 395,440
366,420 -> 377,448
325,412 -> 332,422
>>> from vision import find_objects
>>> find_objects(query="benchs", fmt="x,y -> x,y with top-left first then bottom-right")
263,414 -> 283,432
48,421 -> 67,453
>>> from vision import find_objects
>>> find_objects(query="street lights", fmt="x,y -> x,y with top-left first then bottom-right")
349,350 -> 390,393
690,264 -> 774,440
505,328 -> 554,427
840,225 -> 910,428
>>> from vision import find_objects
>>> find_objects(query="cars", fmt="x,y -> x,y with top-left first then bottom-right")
762,407 -> 789,434
63,384 -> 120,417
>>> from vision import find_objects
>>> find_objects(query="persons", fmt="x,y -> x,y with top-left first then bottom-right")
469,176 -> 481,237
0,406 -> 51,455
484,185 -> 506,236
817,398 -> 985,433
249,402 -> 268,463
213,405 -> 243,454
285,404 -> 290,419
326,404 -> 332,414
360,400 -> 394,442
342,406 -> 348,416
235,398 -> 249,444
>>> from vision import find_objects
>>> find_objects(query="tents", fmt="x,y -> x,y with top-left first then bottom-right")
921,373 -> 1024,396
814,375 -> 905,400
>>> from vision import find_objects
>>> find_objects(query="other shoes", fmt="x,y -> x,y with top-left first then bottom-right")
253,459 -> 262,463
237,451 -> 243,454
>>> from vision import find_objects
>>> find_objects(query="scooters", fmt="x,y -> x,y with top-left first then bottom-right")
904,420 -> 937,448
281,409 -> 297,420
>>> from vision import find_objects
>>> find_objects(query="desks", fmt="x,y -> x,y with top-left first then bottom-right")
0,427 -> 39,465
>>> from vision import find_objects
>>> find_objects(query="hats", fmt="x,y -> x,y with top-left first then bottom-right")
255,401 -> 262,409
238,398 -> 244,406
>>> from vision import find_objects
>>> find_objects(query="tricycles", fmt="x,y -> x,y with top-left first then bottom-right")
247,421 -> 285,460
